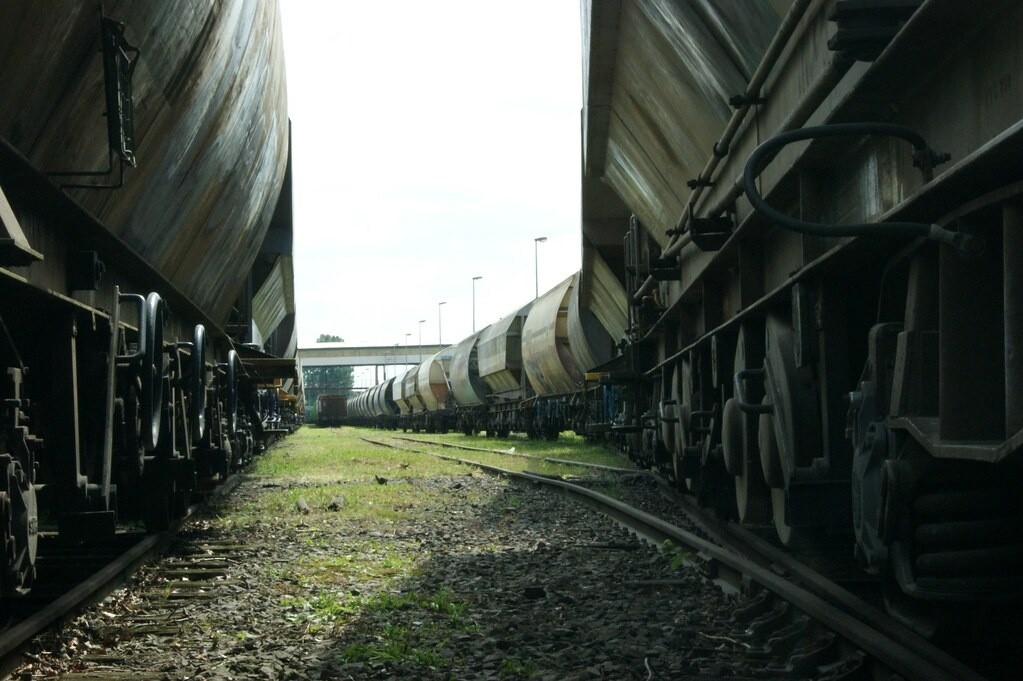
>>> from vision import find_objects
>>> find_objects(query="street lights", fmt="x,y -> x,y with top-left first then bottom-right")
438,301 -> 448,352
535,237 -> 546,298
473,276 -> 482,333
419,319 -> 427,365
393,343 -> 399,378
405,334 -> 411,370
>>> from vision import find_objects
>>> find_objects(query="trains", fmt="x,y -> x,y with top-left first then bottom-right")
306,393 -> 347,430
348,1 -> 1023,681
0,1 -> 306,632
